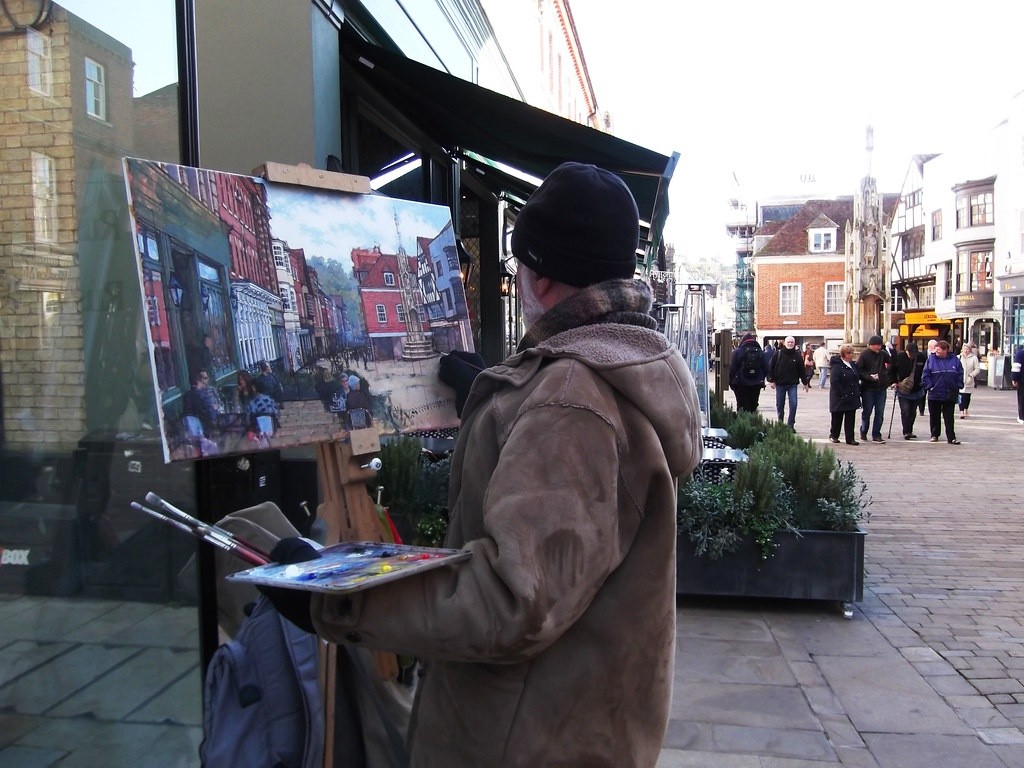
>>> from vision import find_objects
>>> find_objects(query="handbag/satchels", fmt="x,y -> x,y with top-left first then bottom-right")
805,355 -> 814,366
899,377 -> 914,395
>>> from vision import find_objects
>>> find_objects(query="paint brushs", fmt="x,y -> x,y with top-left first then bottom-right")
145,491 -> 272,565
432,348 -> 484,373
193,530 -> 269,569
130,502 -> 206,542
205,521 -> 272,561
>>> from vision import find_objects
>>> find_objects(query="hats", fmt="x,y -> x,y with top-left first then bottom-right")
870,336 -> 885,347
511,159 -> 639,287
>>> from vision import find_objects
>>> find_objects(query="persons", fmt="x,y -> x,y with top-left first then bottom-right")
921,340 -> 964,444
768,336 -> 809,433
948,337 -> 980,418
1010,349 -> 1024,424
699,339 -> 896,390
857,336 -> 892,444
891,343 -> 928,439
729,333 -> 767,414
257,163 -> 703,768
828,344 -> 861,445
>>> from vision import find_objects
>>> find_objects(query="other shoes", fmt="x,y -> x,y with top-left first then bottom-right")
1017,417 -> 1024,425
820,386 -> 827,389
787,425 -> 796,433
859,432 -> 868,441
873,437 -> 886,443
949,438 -> 961,444
961,411 -> 968,419
846,439 -> 859,445
930,436 -> 938,442
830,434 -> 840,443
904,432 -> 917,440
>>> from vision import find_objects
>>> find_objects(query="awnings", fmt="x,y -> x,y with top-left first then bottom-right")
357,39 -> 681,279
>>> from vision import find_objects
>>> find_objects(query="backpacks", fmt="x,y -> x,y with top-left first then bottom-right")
199,587 -> 323,768
741,346 -> 763,380
